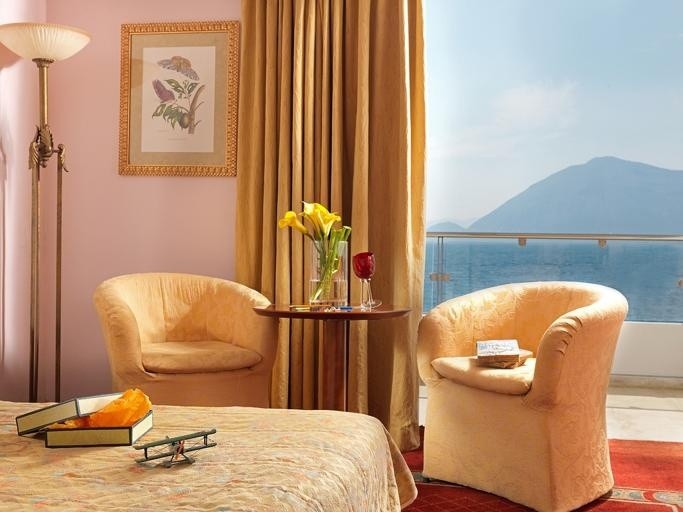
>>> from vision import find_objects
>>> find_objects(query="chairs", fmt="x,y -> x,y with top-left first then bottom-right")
93,272 -> 278,408
416,281 -> 629,512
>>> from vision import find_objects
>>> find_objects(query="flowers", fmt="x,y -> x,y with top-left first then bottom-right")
279,201 -> 351,301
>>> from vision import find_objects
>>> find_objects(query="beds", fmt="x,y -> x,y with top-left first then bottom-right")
0,401 -> 417,512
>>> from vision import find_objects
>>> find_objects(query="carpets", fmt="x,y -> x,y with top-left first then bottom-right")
401,427 -> 683,512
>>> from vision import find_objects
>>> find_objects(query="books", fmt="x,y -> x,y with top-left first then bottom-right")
476,339 -> 534,369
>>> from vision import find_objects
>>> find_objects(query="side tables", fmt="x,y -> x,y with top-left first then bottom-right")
257,301 -> 409,412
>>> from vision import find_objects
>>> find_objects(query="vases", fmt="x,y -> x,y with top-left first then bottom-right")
310,241 -> 348,310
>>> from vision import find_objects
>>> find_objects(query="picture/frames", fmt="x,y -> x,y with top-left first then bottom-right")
120,20 -> 242,174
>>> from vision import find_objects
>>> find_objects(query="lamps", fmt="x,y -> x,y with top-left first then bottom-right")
0,23 -> 93,403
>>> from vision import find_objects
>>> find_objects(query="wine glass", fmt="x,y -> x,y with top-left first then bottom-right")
352,254 -> 374,313
359,253 -> 383,309
